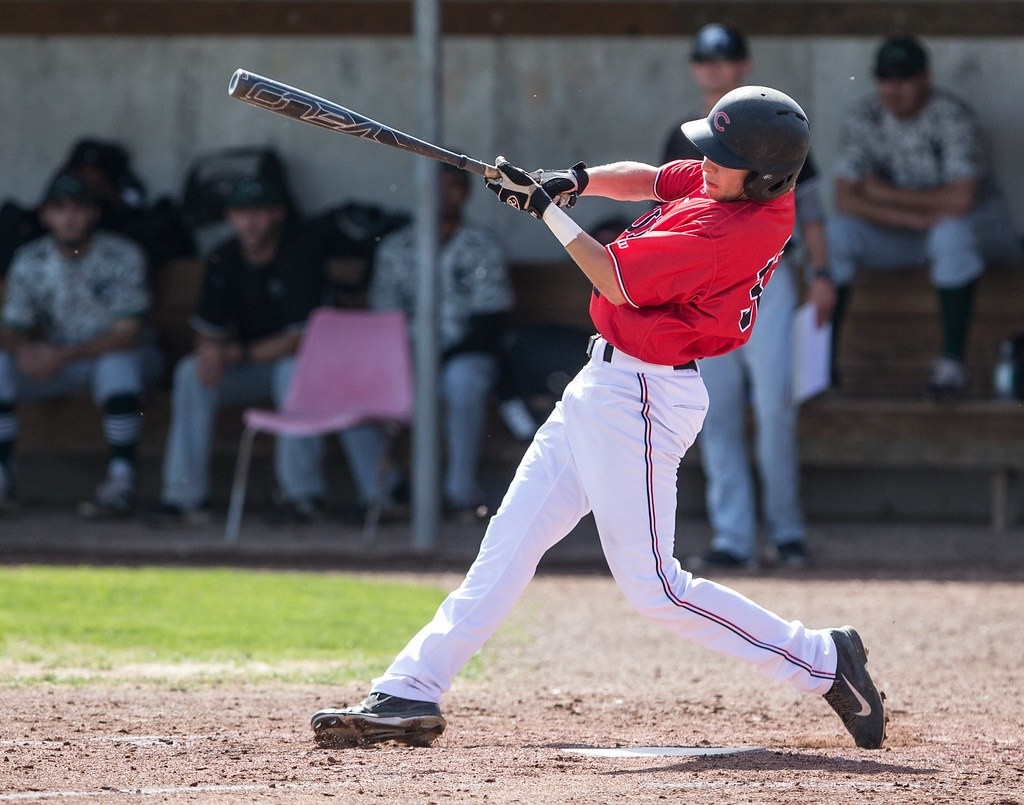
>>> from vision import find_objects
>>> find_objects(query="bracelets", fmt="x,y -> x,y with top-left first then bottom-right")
542,202 -> 582,247
815,270 -> 829,278
240,345 -> 249,364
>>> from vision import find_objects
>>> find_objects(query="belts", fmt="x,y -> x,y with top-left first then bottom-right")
585,335 -> 699,371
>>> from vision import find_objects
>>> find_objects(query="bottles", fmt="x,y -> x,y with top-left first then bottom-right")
993,341 -> 1016,399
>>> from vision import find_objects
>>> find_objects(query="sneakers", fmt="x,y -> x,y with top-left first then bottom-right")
312,691 -> 443,749
826,626 -> 887,751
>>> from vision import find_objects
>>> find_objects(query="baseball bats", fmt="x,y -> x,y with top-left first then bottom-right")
228,68 -> 580,210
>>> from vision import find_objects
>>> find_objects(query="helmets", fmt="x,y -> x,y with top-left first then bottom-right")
681,84 -> 807,199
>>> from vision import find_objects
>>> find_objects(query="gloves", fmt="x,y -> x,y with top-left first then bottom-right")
484,155 -> 553,219
531,161 -> 590,209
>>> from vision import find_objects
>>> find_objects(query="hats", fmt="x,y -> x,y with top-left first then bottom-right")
217,168 -> 283,213
44,170 -> 99,209
691,22 -> 745,65
871,37 -> 929,80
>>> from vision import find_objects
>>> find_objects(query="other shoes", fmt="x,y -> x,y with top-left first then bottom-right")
927,358 -> 967,401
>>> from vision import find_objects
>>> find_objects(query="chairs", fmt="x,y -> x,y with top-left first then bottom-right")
223,306 -> 416,561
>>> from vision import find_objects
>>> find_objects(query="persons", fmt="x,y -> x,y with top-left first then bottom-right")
143,178 -> 325,522
0,172 -> 151,514
659,22 -> 835,574
334,162 -> 517,528
313,86 -> 890,750
506,220 -> 625,414
808,36 -> 1011,395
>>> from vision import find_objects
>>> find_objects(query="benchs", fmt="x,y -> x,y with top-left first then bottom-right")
0,253 -> 414,502
486,253 -> 1024,534
